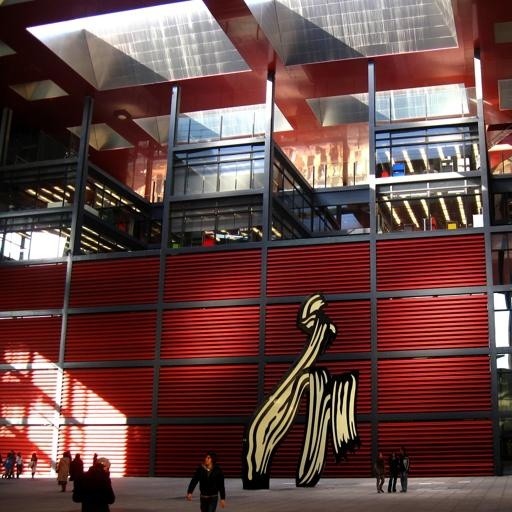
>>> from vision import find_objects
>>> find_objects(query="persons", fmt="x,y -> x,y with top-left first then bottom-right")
387,451 -> 398,493
0,449 -> 115,512
374,451 -> 385,493
187,450 -> 225,512
202,234 -> 215,247
398,448 -> 410,492
